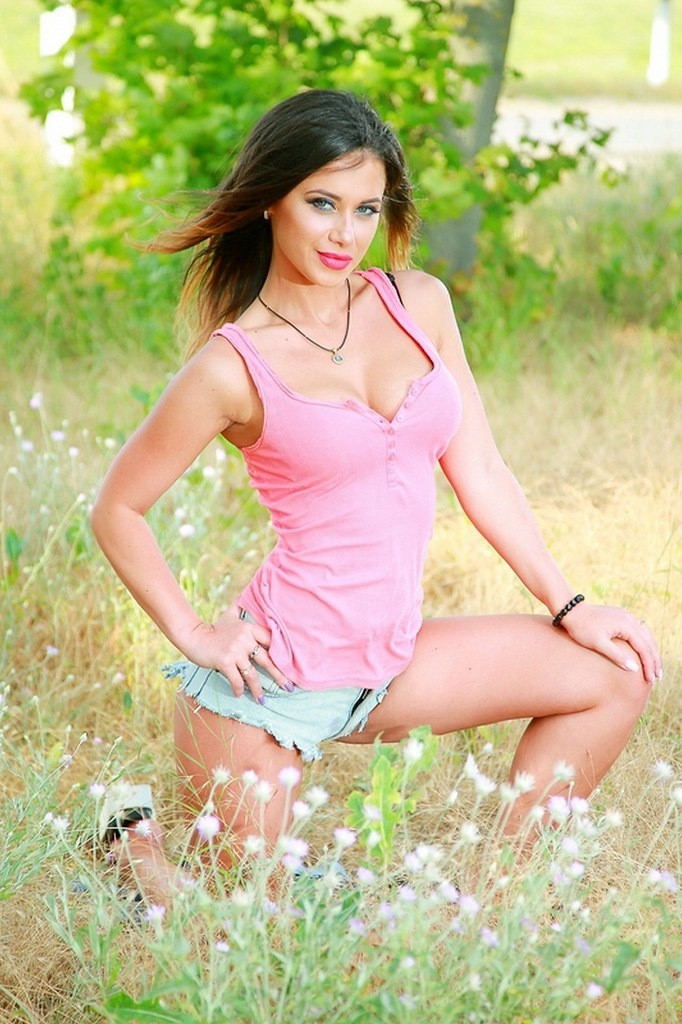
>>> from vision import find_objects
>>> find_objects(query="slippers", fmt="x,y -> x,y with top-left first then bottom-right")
98,785 -> 152,844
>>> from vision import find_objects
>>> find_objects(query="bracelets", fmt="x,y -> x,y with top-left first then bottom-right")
554,592 -> 586,625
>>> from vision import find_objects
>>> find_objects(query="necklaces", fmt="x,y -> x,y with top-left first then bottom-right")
255,278 -> 351,365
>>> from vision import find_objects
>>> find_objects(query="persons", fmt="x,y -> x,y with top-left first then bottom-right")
100,90 -> 662,948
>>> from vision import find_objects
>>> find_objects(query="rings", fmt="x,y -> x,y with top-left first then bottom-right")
240,664 -> 253,679
249,644 -> 260,659
